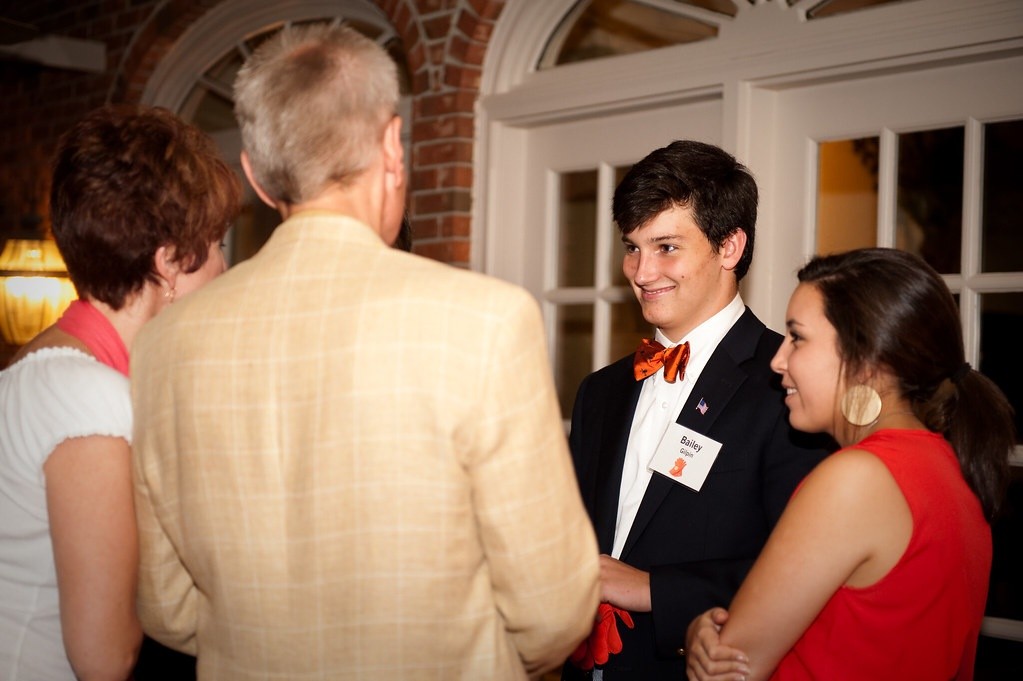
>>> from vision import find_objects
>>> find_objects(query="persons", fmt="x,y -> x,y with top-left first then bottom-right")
131,19 -> 601,681
566,139 -> 839,681
685,246 -> 1014,681
0,103 -> 247,681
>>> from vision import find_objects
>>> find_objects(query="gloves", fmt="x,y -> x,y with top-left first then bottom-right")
574,602 -> 635,670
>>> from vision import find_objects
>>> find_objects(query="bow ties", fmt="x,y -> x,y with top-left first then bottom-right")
633,337 -> 690,383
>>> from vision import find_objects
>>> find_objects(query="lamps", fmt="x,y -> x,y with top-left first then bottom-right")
0,238 -> 79,347
